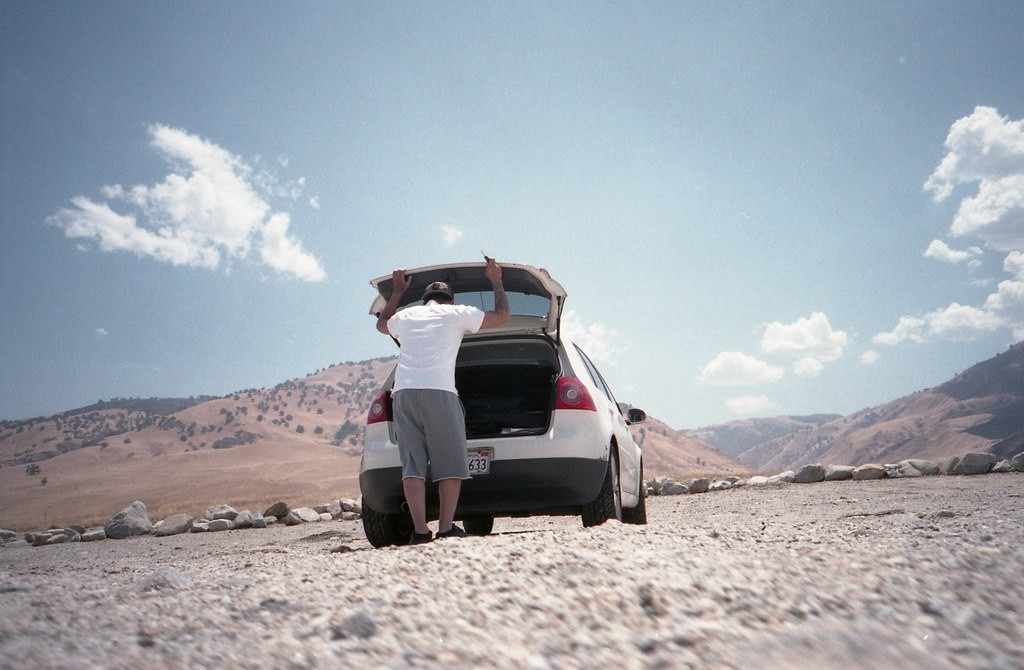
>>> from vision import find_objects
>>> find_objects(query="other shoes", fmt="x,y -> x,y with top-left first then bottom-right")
409,530 -> 432,545
437,522 -> 474,539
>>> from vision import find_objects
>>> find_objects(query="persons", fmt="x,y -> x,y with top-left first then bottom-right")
376,258 -> 511,545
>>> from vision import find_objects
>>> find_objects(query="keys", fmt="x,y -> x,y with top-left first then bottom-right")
481,250 -> 489,262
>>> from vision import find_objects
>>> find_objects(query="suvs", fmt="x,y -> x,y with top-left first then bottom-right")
358,259 -> 648,550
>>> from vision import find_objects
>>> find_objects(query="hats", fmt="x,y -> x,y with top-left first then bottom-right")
422,281 -> 454,303
